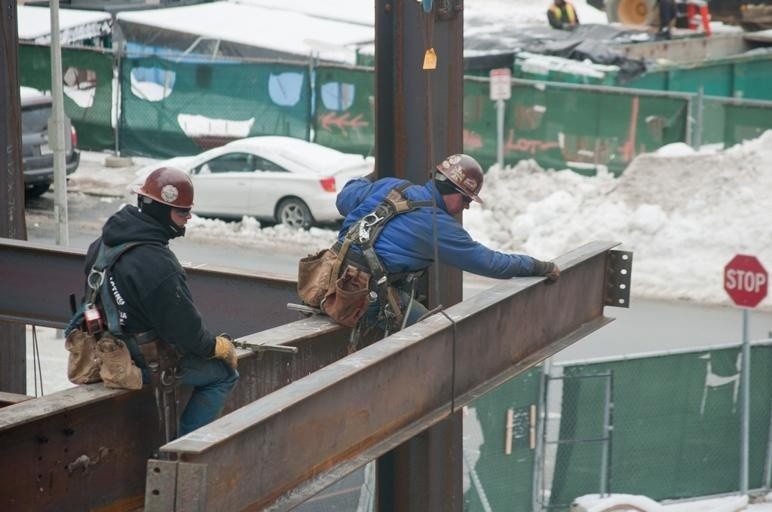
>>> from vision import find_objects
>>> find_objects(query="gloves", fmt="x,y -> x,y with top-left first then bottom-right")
530,257 -> 560,286
210,336 -> 238,369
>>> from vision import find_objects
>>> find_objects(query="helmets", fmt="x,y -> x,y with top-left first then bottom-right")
133,167 -> 193,209
436,154 -> 484,204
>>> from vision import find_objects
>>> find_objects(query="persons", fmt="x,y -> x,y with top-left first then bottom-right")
547,0 -> 579,31
298,153 -> 560,334
81,167 -> 241,438
653,0 -> 679,42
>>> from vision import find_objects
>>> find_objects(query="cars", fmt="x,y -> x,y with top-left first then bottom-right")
125,136 -> 375,231
463,23 -> 670,77
19,85 -> 80,200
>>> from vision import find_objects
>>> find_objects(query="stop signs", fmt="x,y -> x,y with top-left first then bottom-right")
721,252 -> 769,310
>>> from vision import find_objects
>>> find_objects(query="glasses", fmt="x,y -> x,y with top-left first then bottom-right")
174,209 -> 191,216
455,188 -> 472,203
